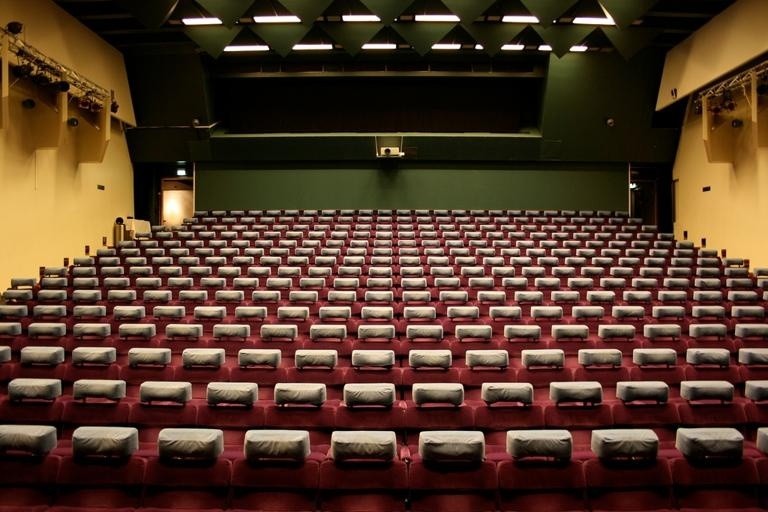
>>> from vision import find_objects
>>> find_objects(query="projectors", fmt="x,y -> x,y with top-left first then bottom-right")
380,146 -> 399,156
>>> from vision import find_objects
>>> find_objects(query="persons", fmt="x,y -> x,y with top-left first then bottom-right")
383,148 -> 390,155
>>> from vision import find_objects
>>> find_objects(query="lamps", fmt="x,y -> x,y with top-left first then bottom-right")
182,15 -> 616,52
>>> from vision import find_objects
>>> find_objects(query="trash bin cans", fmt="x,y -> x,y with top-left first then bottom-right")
115,224 -> 124,249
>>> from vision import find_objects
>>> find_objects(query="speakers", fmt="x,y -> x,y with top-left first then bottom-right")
135,162 -> 160,225
656,180 -> 672,232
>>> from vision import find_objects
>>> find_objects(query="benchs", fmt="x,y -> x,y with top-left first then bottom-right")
0,210 -> 768,512
